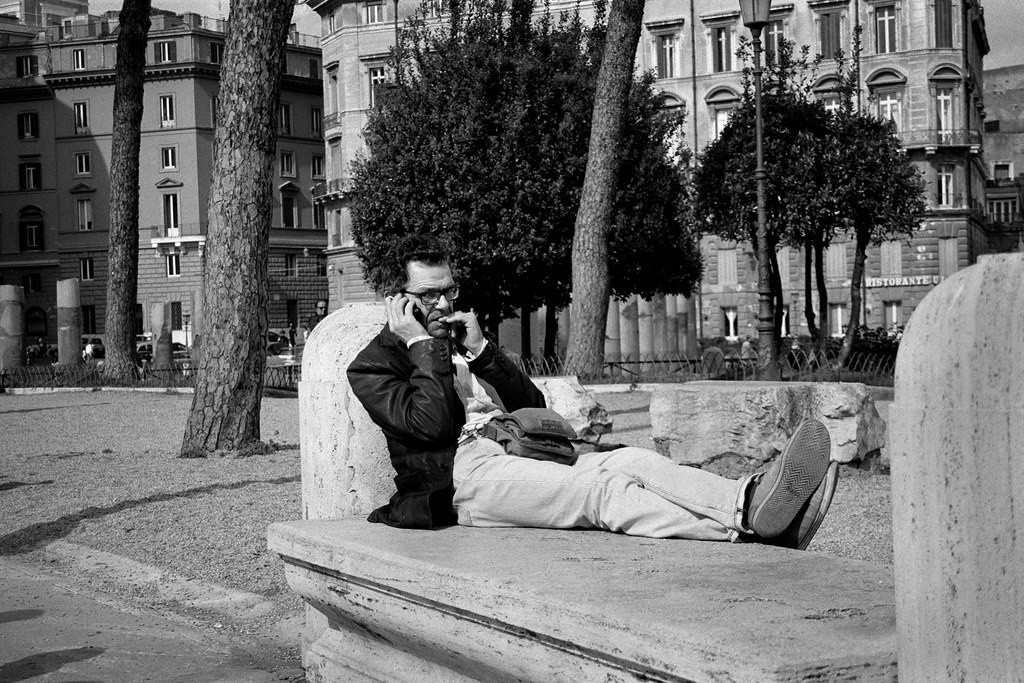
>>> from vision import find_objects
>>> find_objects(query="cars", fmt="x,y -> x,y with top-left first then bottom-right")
136,334 -> 191,365
81,337 -> 105,353
267,332 -> 289,354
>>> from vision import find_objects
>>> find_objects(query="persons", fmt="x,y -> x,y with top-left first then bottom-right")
741,335 -> 753,358
701,335 -> 726,380
787,339 -> 805,370
349,236 -> 839,551
308,299 -> 328,332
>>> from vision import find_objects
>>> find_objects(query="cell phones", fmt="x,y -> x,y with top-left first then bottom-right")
389,295 -> 424,325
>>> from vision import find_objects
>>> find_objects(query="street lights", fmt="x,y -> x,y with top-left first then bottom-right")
736,0 -> 780,378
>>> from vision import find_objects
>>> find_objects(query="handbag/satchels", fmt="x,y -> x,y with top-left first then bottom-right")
482,408 -> 579,465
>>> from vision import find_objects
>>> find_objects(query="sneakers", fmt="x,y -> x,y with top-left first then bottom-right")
747,419 -> 832,537
778,461 -> 839,551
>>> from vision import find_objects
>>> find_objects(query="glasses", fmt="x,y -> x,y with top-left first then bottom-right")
397,283 -> 460,306
316,307 -> 328,311
